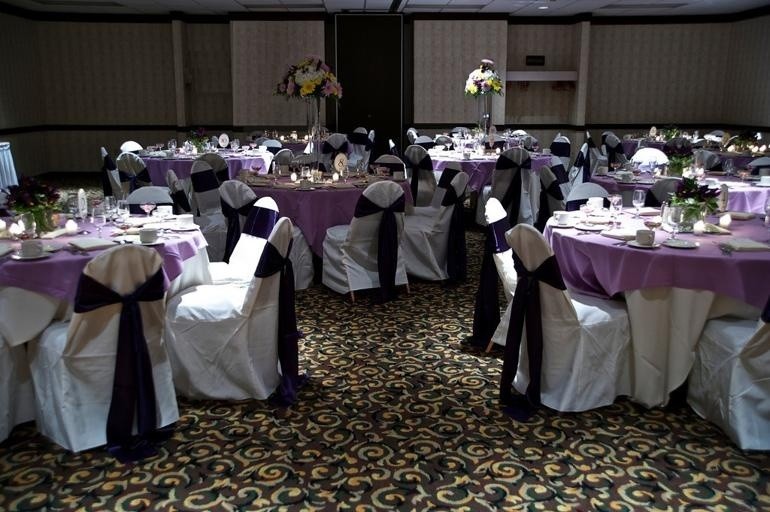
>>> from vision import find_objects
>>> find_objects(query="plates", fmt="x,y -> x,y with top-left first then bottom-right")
662,239 -> 700,249
43,242 -> 66,252
171,224 -> 200,231
752,182 -> 770,187
600,230 -> 636,239
164,215 -> 177,220
550,221 -> 574,228
628,239 -> 662,248
11,252 -> 50,260
747,174 -> 760,181
113,216 -> 170,246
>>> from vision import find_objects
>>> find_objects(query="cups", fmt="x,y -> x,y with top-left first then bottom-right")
21,241 -> 43,256
176,213 -> 194,229
597,166 -> 608,176
635,230 -> 656,245
758,169 -> 770,186
553,211 -> 571,225
157,205 -> 172,217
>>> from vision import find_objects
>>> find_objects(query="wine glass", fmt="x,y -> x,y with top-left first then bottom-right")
433,125 -> 551,162
575,189 -> 647,231
764,197 -> 770,228
139,200 -> 157,221
67,187 -> 131,238
139,130 -> 298,161
681,130 -> 700,143
608,155 -> 748,186
7,209 -> 18,223
238,160 -> 404,190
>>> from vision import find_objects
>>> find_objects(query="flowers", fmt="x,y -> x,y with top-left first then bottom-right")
464,60 -> 504,96
273,54 -> 342,100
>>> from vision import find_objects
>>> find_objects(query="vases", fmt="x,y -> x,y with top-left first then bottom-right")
479,96 -> 490,154
305,96 -> 319,178
